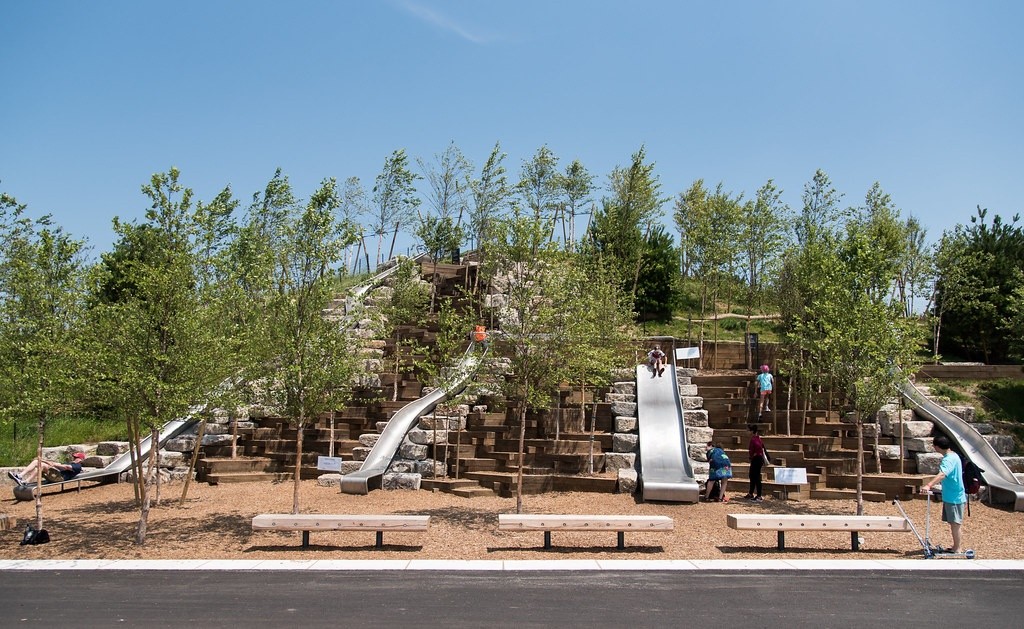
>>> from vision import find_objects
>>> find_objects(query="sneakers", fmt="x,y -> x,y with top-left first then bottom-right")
750,496 -> 763,502
743,494 -> 754,500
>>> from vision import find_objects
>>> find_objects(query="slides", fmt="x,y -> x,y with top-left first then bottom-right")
13,254 -> 423,503
339,336 -> 490,496
881,359 -> 1023,511
632,348 -> 701,502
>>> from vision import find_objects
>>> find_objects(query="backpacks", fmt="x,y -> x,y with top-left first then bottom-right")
954,452 -> 985,494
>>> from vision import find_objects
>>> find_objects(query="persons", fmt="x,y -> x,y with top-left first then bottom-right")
647,344 -> 668,377
702,441 -> 732,502
8,452 -> 85,486
742,425 -> 770,502
754,365 -> 774,418
473,317 -> 487,353
919,435 -> 967,555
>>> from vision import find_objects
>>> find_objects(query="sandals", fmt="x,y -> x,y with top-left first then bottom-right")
702,497 -> 709,502
718,497 -> 723,502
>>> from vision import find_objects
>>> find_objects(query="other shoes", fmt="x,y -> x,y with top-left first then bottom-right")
766,409 -> 771,411
8,471 -> 26,486
758,414 -> 762,421
653,368 -> 656,377
945,547 -> 955,554
658,368 -> 664,377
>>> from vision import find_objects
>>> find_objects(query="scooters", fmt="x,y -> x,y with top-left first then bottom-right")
920,490 -> 976,559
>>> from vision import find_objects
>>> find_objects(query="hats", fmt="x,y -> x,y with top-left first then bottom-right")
760,364 -> 769,372
72,453 -> 85,459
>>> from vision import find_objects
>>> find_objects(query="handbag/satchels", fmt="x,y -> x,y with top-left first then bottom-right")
762,443 -> 771,466
20,523 -> 50,546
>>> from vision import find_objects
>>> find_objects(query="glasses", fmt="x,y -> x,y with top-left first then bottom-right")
747,430 -> 752,432
